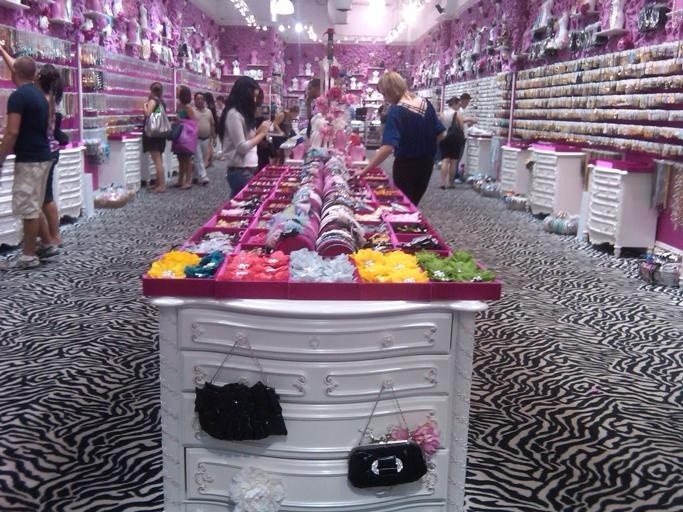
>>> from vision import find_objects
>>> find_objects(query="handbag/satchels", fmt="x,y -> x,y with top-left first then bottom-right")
172,119 -> 199,154
53,112 -> 68,145
346,382 -> 427,488
446,112 -> 465,147
166,122 -> 183,140
143,103 -> 172,138
195,334 -> 288,441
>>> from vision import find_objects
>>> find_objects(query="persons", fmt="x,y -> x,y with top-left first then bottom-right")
169,85 -> 199,189
0,45 -> 65,249
304,78 -> 320,139
452,92 -> 479,185
349,69 -> 445,209
141,81 -> 167,194
187,90 -> 300,190
437,96 -> 465,189
0,56 -> 61,272
219,76 -> 271,197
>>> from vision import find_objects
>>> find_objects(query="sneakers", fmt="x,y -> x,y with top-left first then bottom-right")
37,244 -> 59,260
200,176 -> 209,184
0,250 -> 42,270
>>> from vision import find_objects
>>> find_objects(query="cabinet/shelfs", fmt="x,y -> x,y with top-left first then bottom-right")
527,148 -> 587,220
51,147 -> 88,227
98,133 -> 180,194
583,163 -> 658,258
353,124 -> 384,149
499,146 -> 528,197
146,296 -> 489,512
463,137 -> 492,183
0,154 -> 26,250
